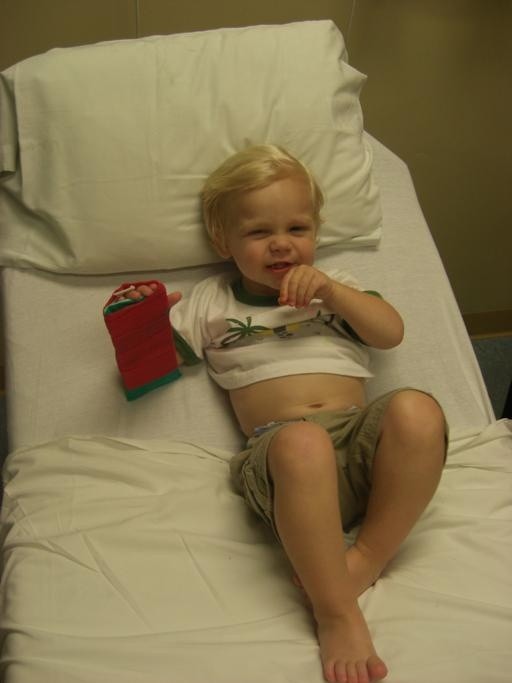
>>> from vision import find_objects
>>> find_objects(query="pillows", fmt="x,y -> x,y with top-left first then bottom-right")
0,19 -> 384,275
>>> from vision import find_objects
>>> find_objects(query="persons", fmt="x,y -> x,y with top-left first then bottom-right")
109,142 -> 448,682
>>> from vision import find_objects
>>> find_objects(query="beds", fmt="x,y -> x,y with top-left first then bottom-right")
0,129 -> 512,683
